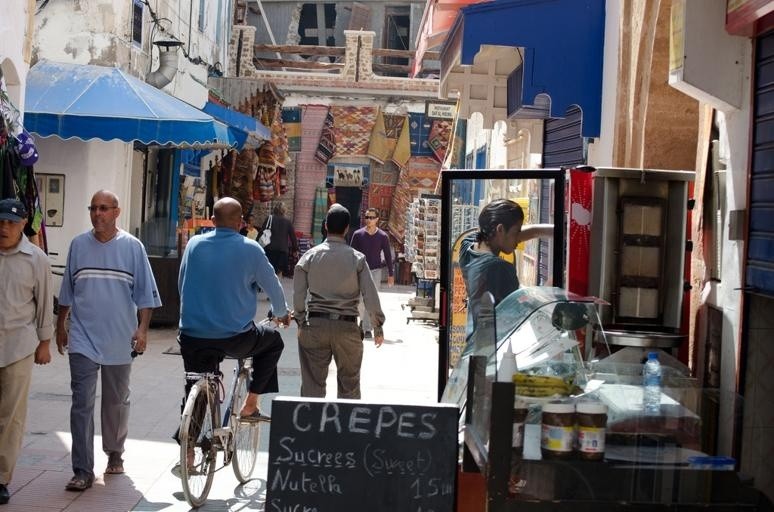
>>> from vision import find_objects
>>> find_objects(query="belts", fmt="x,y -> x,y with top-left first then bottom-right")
307,311 -> 357,321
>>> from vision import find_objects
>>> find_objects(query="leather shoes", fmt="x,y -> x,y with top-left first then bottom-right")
0,484 -> 9,505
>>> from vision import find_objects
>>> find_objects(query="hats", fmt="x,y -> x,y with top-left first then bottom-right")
0,198 -> 29,224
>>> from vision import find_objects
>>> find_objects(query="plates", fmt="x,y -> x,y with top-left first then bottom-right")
515,393 -> 560,402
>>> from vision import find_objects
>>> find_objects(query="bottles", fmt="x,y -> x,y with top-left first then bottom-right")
643,352 -> 661,411
499,335 -> 518,383
522,402 -> 609,460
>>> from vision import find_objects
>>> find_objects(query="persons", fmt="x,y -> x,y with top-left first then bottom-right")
349,207 -> 395,339
321,202 -> 342,243
1,199 -> 56,505
459,198 -> 555,382
239,215 -> 263,240
170,197 -> 291,471
56,189 -> 163,492
255,202 -> 302,302
293,206 -> 385,398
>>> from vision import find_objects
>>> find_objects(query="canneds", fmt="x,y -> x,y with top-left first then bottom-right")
541,403 -> 575,460
572,402 -> 609,460
511,402 -> 527,449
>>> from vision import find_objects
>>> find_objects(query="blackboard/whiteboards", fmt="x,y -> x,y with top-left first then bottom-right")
264,396 -> 460,512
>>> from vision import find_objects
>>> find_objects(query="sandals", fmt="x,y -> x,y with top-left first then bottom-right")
65,476 -> 93,490
106,452 -> 124,474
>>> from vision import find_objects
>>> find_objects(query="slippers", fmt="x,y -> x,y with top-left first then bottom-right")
240,408 -> 272,423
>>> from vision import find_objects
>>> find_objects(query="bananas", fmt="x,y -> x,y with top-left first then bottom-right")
512,373 -> 584,398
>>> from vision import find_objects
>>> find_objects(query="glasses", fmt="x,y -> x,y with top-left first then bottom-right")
88,205 -> 117,212
365,216 -> 378,220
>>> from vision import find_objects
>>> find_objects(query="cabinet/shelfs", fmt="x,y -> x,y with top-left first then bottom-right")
460,354 -> 740,512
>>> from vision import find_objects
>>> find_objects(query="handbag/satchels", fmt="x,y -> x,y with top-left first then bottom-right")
258,228 -> 271,247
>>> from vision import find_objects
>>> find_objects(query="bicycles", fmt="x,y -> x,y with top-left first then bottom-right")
176,312 -> 283,510
